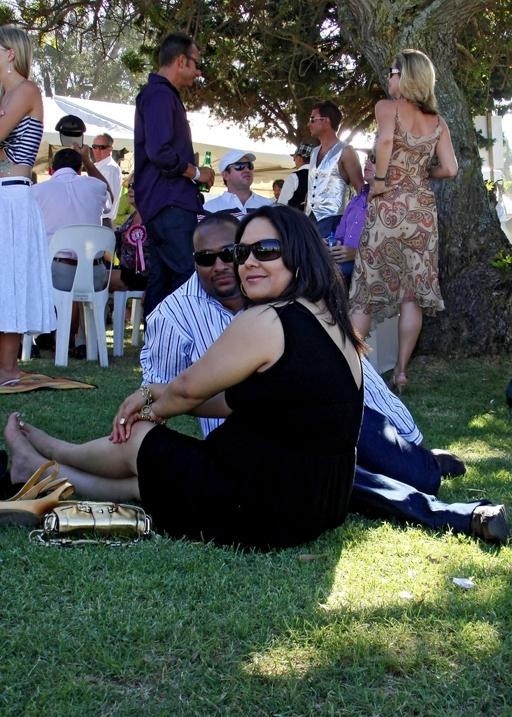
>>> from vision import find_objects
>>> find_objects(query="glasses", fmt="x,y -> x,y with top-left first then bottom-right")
187,55 -> 203,71
191,241 -> 235,267
92,143 -> 112,150
308,115 -> 328,123
225,161 -> 255,171
233,239 -> 286,266
388,67 -> 401,80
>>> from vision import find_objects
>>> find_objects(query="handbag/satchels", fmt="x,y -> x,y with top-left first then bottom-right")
42,497 -> 152,543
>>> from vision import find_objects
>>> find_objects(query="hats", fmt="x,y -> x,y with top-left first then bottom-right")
55,113 -> 86,149
218,149 -> 255,176
291,143 -> 315,158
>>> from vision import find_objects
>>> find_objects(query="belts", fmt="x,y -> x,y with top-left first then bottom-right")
2,179 -> 31,187
52,255 -> 104,266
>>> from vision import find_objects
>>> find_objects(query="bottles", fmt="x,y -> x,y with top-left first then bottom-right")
198,149 -> 212,192
327,230 -> 336,251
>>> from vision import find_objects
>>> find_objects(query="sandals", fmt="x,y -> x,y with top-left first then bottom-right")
0,459 -> 76,521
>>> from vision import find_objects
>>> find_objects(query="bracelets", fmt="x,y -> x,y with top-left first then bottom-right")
139,384 -> 154,414
141,409 -> 167,425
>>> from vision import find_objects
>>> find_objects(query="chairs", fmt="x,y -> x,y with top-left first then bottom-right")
109,290 -> 145,357
20,222 -> 117,369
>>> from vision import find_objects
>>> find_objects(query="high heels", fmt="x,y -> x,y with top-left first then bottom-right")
386,368 -> 408,396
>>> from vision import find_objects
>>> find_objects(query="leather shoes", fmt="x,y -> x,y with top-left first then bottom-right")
68,343 -> 86,360
430,447 -> 466,480
29,344 -> 41,360
470,499 -> 509,542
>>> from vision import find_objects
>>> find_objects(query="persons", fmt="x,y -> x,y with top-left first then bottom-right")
2,25 -> 459,396
138,210 -> 508,544
3,203 -> 365,551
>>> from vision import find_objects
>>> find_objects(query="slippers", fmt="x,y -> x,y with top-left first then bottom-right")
0,375 -> 27,391
